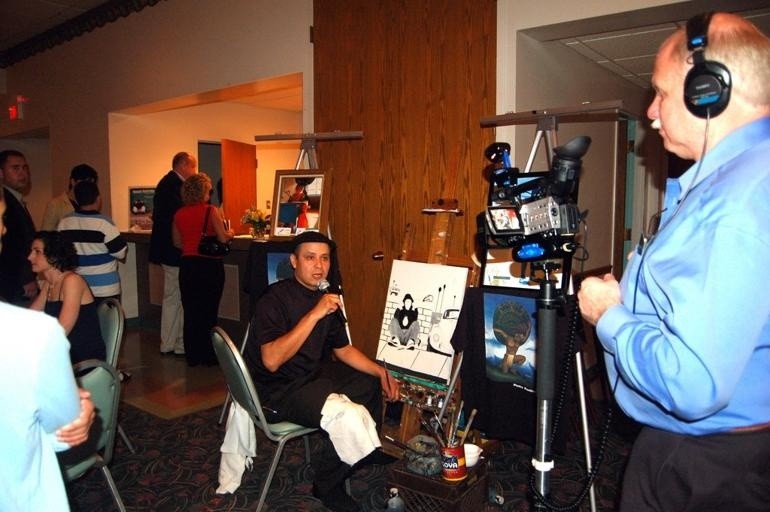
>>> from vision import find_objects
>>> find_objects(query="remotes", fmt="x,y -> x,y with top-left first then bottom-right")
317,278 -> 349,326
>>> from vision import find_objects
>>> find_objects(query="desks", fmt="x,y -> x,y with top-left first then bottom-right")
385,433 -> 499,512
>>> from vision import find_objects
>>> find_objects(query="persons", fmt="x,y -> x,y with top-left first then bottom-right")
388,294 -> 420,350
242,231 -> 401,512
170,175 -> 235,367
500,212 -> 510,228
493,321 -> 532,374
0,172 -> 98,512
575,10 -> 770,511
0,148 -> 135,385
150,152 -> 199,359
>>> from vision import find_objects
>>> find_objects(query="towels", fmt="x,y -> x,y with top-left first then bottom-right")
319,391 -> 384,466
214,403 -> 257,495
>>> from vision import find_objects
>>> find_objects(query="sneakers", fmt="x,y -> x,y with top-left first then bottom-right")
406,343 -> 414,350
388,339 -> 400,347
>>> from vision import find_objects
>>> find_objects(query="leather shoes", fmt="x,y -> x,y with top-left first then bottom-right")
311,487 -> 362,512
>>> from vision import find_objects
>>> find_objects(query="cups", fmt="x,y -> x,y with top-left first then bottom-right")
463,442 -> 481,469
440,445 -> 469,481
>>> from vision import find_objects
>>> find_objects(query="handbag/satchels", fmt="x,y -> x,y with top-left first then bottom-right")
197,206 -> 230,257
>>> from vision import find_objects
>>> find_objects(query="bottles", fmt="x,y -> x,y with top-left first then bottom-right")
385,486 -> 405,512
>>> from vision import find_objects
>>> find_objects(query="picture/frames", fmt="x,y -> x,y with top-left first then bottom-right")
269,168 -> 333,241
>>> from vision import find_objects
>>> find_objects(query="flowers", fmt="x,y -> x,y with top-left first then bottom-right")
239,207 -> 271,227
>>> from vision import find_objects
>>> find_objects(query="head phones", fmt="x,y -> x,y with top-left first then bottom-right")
684,11 -> 732,120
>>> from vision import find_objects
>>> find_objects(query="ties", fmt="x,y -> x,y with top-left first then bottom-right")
22,202 -> 30,219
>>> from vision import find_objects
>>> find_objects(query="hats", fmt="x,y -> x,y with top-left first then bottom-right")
403,294 -> 414,302
292,231 -> 337,254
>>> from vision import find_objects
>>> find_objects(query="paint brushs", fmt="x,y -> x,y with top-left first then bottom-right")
421,400 -> 478,447
382,359 -> 392,391
384,434 -> 419,454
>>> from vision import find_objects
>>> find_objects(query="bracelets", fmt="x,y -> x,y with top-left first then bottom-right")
228,231 -> 235,236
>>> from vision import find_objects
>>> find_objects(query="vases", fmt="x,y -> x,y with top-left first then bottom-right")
249,228 -> 266,239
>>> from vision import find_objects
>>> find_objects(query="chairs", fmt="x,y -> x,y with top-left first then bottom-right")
26,362 -> 128,510
210,325 -> 353,512
74,298 -> 136,466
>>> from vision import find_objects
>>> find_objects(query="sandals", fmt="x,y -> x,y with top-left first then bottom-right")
116,371 -> 133,383
